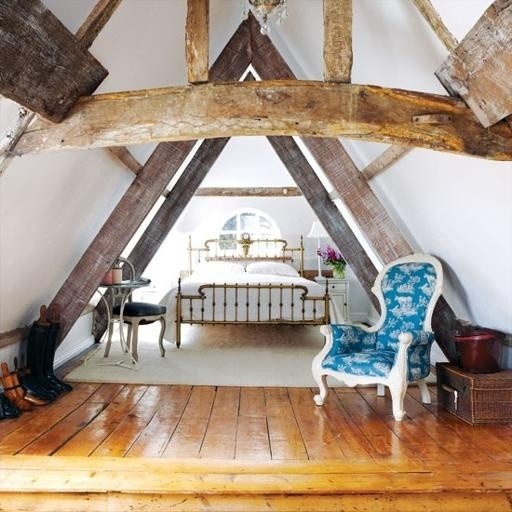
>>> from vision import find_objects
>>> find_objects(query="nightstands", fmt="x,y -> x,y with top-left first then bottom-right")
314,278 -> 352,325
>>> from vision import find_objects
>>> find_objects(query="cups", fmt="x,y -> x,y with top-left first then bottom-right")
112,268 -> 122,285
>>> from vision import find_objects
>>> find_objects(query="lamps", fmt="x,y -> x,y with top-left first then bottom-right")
241,0 -> 289,36
307,221 -> 330,280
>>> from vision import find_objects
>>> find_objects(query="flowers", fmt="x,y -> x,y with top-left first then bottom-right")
317,244 -> 347,279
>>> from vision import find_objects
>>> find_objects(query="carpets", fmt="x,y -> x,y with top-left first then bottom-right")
62,322 -> 437,388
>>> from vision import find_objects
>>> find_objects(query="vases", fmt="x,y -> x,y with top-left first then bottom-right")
332,268 -> 345,279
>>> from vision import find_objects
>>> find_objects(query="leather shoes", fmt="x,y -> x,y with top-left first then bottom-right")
0,362 -> 49,420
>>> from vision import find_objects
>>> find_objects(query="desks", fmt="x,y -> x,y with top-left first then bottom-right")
84,280 -> 150,371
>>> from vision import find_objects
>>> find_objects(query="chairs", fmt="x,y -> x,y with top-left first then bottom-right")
311,253 -> 444,422
104,257 -> 167,364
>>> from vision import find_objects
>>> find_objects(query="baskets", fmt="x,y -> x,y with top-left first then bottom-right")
434,360 -> 511,426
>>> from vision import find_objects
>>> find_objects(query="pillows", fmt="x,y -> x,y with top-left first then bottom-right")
192,261 -> 300,276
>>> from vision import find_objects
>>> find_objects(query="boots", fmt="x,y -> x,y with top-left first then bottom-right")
39,319 -> 73,392
27,322 -> 60,393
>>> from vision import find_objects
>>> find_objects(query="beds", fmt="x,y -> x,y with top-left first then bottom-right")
157,232 -> 345,349
